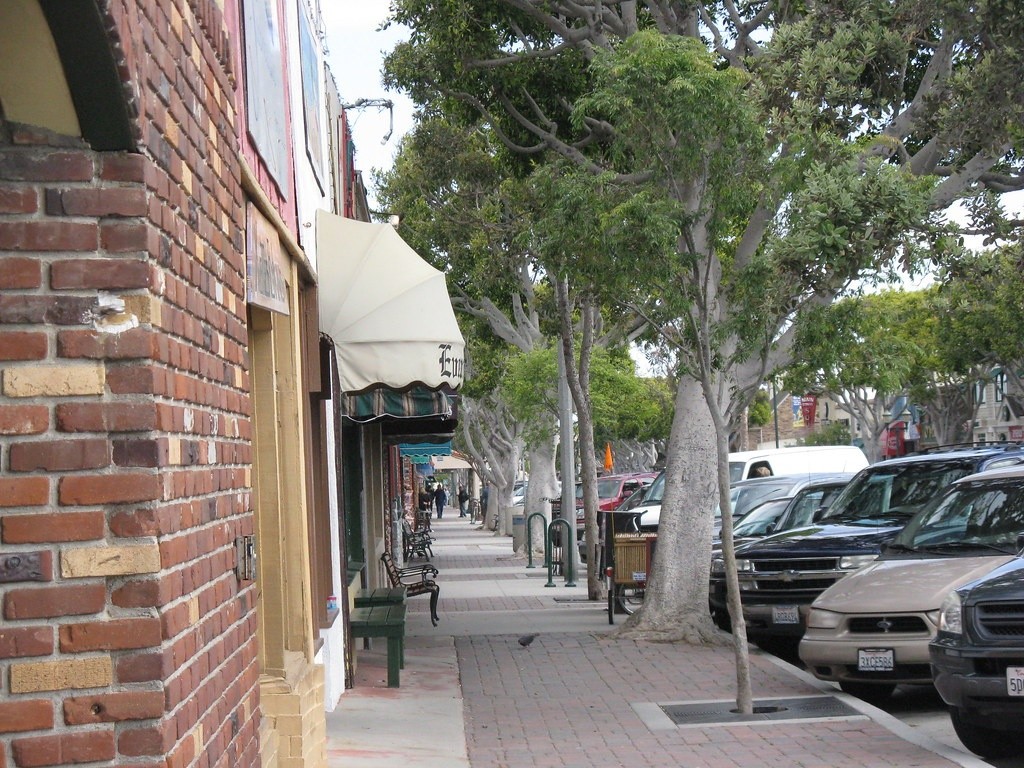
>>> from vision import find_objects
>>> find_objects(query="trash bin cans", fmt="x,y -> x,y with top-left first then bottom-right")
512,514 -> 526,553
596,511 -> 642,580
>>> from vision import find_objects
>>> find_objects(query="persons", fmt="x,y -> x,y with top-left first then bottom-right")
751,467 -> 771,477
418,481 -> 447,521
480,485 -> 489,517
457,486 -> 469,518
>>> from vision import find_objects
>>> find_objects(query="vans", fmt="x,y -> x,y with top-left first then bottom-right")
627,444 -> 871,537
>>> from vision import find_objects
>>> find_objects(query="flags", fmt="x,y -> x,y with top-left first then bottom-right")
604,445 -> 613,471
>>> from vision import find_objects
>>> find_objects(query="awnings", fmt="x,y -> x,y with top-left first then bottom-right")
315,209 -> 465,465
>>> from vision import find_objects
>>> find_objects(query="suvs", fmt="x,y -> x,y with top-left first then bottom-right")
706,441 -> 1024,660
928,540 -> 1024,755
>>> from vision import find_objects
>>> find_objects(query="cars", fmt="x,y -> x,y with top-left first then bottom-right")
799,459 -> 1024,700
512,471 -> 662,541
705,473 -> 859,560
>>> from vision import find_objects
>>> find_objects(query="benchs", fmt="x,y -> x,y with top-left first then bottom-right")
402,508 -> 436,561
382,551 -> 439,626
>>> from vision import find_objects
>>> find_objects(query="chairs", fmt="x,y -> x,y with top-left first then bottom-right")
813,493 -> 839,523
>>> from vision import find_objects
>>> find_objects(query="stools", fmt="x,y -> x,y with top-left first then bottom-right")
354,587 -> 408,650
352,605 -> 407,686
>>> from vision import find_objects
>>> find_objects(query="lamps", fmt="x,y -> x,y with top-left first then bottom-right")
343,98 -> 393,145
